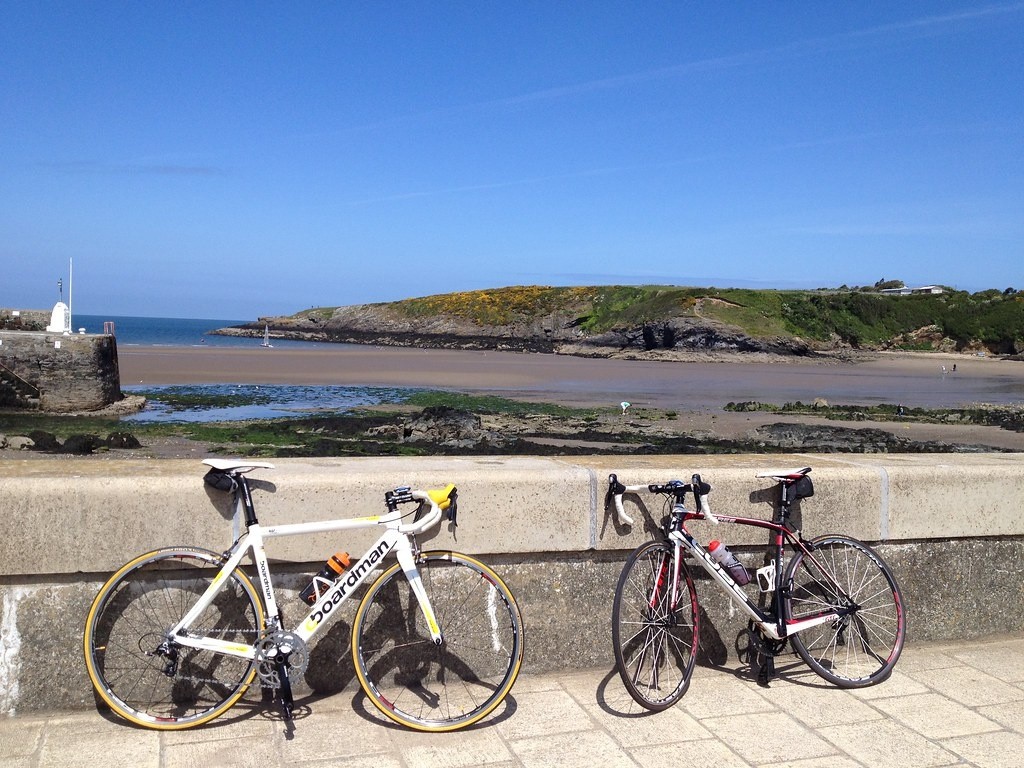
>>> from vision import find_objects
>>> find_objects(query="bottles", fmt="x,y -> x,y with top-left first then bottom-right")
299,552 -> 351,608
707,541 -> 750,585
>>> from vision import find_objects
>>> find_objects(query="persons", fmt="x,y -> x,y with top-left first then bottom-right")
953,363 -> 956,371
896,404 -> 903,417
620,401 -> 632,416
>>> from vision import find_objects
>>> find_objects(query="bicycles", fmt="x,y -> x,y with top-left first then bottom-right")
84,459 -> 525,733
604,467 -> 911,711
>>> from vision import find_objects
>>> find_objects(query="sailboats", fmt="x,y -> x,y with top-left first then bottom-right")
260,325 -> 274,348
